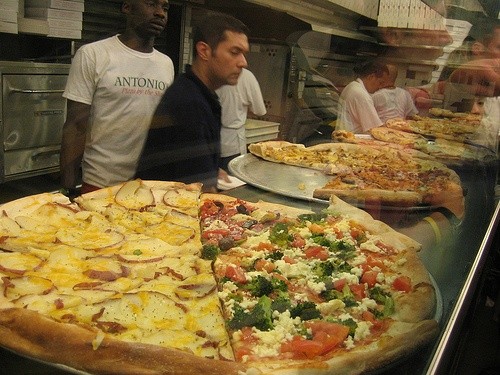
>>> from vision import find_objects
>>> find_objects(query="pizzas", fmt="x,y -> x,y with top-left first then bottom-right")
0,181 -> 439,375
248,108 -> 486,204
